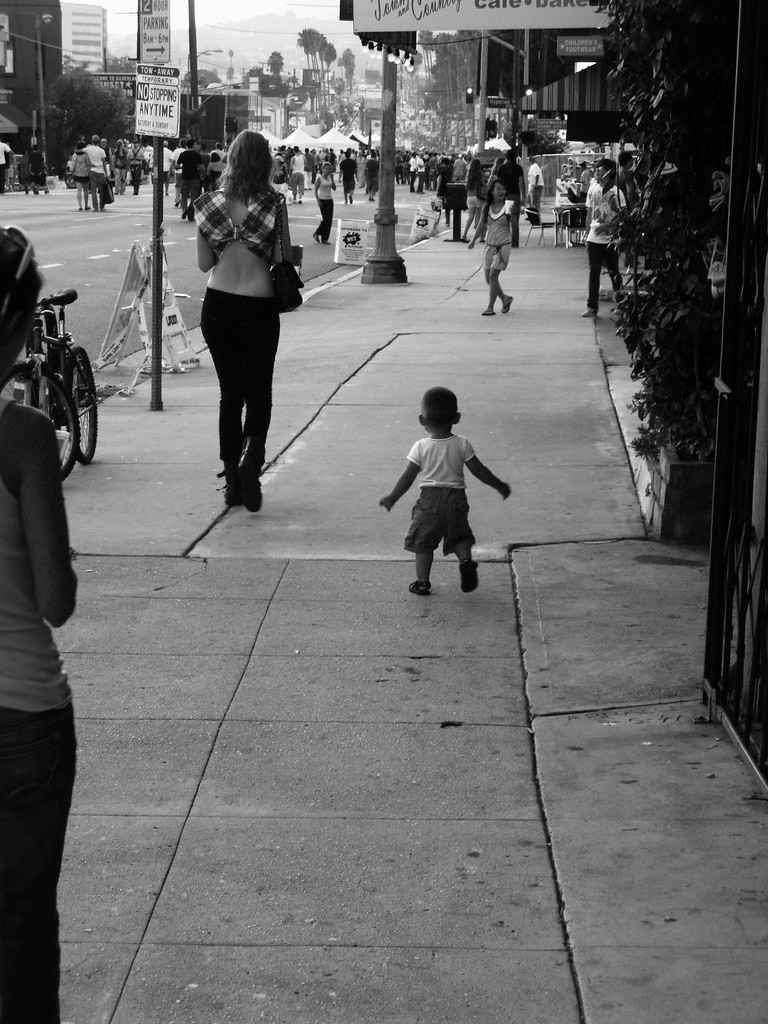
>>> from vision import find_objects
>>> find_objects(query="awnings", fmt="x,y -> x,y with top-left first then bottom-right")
520,61 -> 635,143
0,105 -> 41,134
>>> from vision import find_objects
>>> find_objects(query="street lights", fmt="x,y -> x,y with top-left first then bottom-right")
186,51 -> 211,135
34,12 -> 54,177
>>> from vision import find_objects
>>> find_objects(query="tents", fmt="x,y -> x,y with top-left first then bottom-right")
259,126 -> 512,172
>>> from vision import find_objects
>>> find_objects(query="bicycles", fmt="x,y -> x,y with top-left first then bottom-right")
0,286 -> 99,484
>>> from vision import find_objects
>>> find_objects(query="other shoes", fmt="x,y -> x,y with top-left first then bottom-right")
313,233 -> 320,242
293,198 -> 302,204
216,467 -> 245,508
369,198 -> 375,202
349,195 -> 353,204
175,202 -> 179,207
181,207 -> 189,219
240,463 -> 264,512
85,206 -> 91,210
79,207 -> 83,210
321,240 -> 331,245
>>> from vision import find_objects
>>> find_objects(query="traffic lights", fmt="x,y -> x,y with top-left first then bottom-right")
466,87 -> 473,103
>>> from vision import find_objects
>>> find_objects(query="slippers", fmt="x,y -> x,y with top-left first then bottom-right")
482,309 -> 496,316
502,296 -> 514,313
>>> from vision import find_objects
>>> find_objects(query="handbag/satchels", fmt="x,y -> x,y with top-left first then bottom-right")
271,255 -> 303,314
476,182 -> 490,200
554,177 -> 583,206
103,178 -> 114,204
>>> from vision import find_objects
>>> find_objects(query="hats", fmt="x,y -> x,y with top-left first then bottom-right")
93,134 -> 100,142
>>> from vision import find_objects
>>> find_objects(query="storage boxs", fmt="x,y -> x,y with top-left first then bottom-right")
334,219 -> 371,265
410,207 -> 440,239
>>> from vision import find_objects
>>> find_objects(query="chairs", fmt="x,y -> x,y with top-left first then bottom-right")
525,207 -> 588,250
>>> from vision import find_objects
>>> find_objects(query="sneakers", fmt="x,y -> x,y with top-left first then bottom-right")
580,306 -> 598,317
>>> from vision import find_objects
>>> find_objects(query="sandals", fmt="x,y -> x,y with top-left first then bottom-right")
407,580 -> 432,596
459,557 -> 479,593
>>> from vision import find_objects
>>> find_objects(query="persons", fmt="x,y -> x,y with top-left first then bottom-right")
378,387 -> 512,595
192,129 -> 293,513
0,135 -> 640,317
0,226 -> 77,1024
